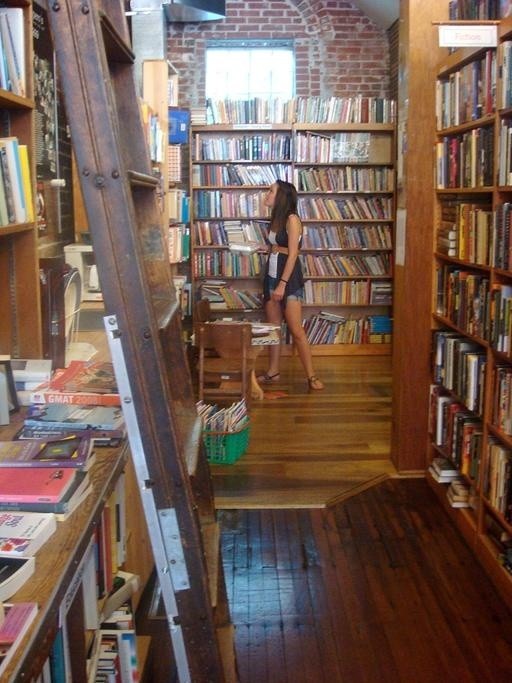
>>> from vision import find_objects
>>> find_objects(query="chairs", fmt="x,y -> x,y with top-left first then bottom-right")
197,321 -> 252,409
190,295 -> 211,331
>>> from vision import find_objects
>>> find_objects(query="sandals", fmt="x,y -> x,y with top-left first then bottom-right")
308,375 -> 325,390
255,370 -> 281,385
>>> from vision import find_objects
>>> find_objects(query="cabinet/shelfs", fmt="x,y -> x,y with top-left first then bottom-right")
140,59 -> 191,278
431,0 -> 510,581
190,121 -> 397,355
1,0 -> 160,681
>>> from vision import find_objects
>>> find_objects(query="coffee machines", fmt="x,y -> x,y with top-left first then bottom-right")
64,242 -> 105,301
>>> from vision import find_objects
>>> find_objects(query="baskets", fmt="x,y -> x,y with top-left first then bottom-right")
200,415 -> 255,467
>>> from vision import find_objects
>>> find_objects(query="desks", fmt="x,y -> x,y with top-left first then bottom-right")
192,324 -> 281,399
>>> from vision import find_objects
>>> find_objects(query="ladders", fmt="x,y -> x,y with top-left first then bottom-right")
34,0 -> 248,683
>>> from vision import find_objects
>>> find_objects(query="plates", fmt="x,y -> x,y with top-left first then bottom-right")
63,264 -> 83,343
